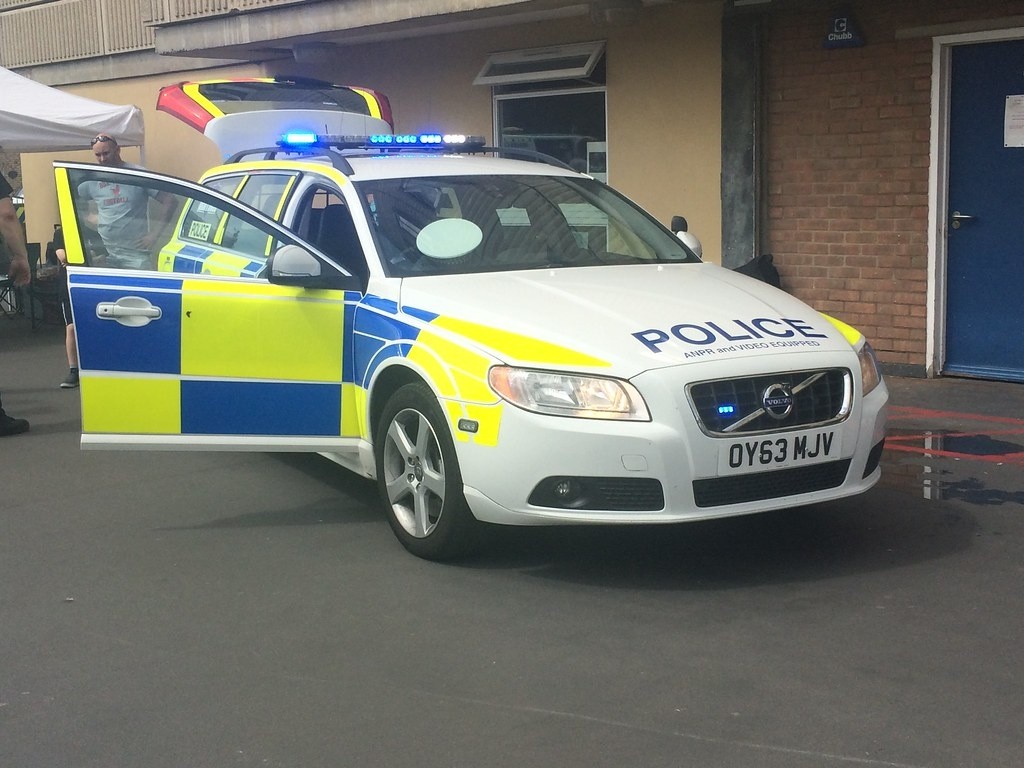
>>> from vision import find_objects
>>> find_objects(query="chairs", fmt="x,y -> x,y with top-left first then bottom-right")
46,242 -> 58,267
315,202 -> 361,268
25,243 -> 42,268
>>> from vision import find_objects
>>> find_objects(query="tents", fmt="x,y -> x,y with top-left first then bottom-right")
0,63 -> 148,173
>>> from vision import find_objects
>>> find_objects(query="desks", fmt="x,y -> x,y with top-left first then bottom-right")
0,274 -> 24,321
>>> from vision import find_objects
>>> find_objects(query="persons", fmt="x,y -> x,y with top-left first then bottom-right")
0,173 -> 31,436
53,199 -> 110,388
76,131 -> 179,274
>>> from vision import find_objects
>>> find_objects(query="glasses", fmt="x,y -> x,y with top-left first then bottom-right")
90,135 -> 117,146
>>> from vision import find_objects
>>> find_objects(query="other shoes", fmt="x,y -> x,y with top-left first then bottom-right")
60,367 -> 79,388
0,408 -> 29,435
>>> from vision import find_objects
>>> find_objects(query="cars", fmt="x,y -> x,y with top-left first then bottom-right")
48,75 -> 890,561
